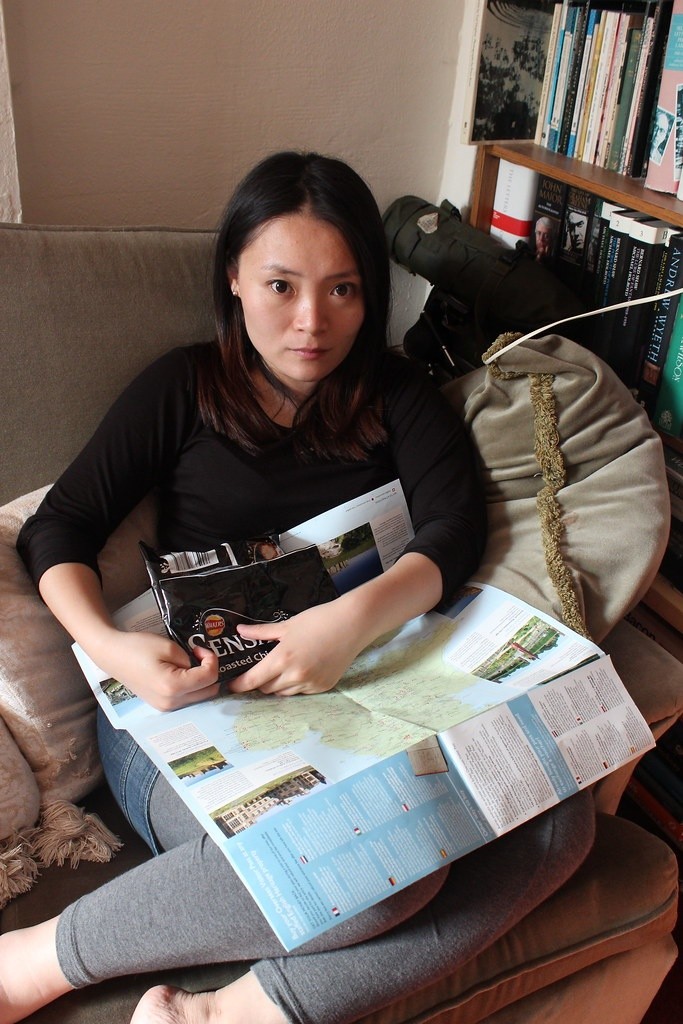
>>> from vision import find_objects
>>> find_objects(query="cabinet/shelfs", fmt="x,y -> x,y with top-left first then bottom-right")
470,137 -> 683,602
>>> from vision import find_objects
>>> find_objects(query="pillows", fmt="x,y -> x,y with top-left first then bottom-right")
0,460 -> 163,923
443,328 -> 672,653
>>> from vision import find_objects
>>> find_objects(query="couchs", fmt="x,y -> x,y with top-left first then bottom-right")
0,216 -> 683,1024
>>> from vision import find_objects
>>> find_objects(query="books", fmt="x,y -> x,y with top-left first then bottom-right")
534,0 -> 683,200
489,157 -> 683,520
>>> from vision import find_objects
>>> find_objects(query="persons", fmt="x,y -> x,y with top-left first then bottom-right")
534,216 -> 556,261
567,212 -> 587,254
589,224 -> 600,256
0,152 -> 596,1024
649,113 -> 668,162
